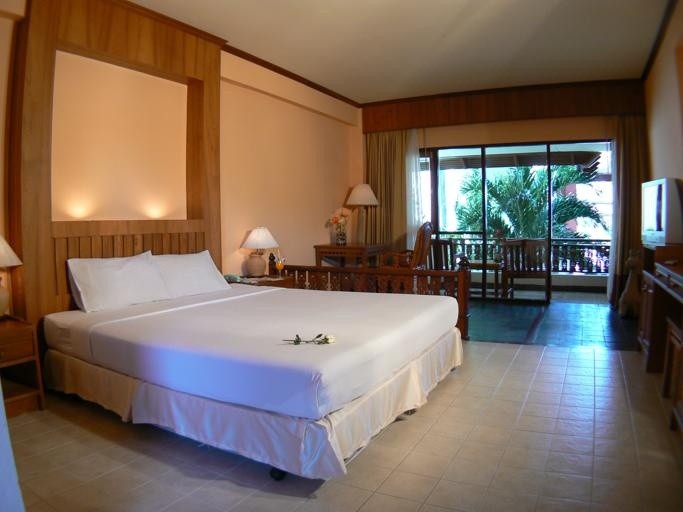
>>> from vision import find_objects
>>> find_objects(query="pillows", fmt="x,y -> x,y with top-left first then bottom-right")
66,248 -> 231,314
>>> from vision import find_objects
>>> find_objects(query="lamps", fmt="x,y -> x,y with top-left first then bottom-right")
346,184 -> 379,244
240,226 -> 279,277
0,234 -> 22,316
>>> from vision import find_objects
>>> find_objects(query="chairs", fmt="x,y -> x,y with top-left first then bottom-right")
378,222 -> 551,306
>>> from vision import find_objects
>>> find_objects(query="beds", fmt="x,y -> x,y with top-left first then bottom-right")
41,281 -> 462,481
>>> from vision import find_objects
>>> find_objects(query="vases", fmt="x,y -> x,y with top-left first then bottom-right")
335,233 -> 346,245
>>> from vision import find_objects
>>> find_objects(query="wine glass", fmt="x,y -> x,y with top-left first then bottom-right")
274,259 -> 285,280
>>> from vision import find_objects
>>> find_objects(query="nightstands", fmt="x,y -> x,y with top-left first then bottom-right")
0,315 -> 46,417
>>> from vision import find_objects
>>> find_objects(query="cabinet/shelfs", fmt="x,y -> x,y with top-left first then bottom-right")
635,242 -> 682,374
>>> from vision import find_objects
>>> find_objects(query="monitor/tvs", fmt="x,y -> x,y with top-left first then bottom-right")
640,176 -> 683,245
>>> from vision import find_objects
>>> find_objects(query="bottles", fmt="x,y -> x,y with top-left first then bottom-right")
269,252 -> 276,275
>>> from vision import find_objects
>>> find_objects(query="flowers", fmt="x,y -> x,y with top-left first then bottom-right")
281,332 -> 336,345
331,207 -> 352,232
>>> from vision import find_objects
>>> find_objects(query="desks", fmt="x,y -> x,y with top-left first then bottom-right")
312,243 -> 385,268
